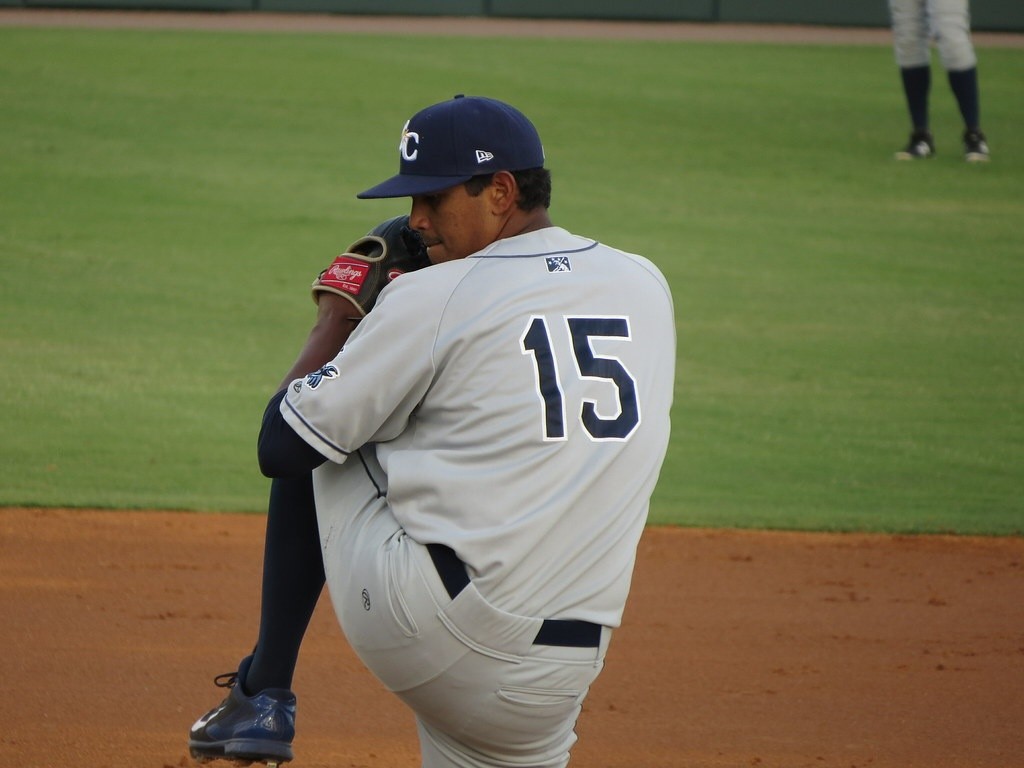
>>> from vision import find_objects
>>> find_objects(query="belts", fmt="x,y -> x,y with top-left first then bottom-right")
424,543 -> 600,649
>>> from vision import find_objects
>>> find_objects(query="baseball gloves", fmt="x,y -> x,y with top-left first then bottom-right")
309,216 -> 429,320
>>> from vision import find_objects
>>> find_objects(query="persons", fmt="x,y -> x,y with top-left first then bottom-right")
186,97 -> 677,768
888,0 -> 996,164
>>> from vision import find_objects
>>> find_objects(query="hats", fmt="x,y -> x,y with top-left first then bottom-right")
356,94 -> 545,199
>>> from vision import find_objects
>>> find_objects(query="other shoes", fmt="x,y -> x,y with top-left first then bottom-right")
965,132 -> 991,163
895,132 -> 933,162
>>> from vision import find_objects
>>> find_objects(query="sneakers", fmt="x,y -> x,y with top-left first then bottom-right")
188,652 -> 296,768
